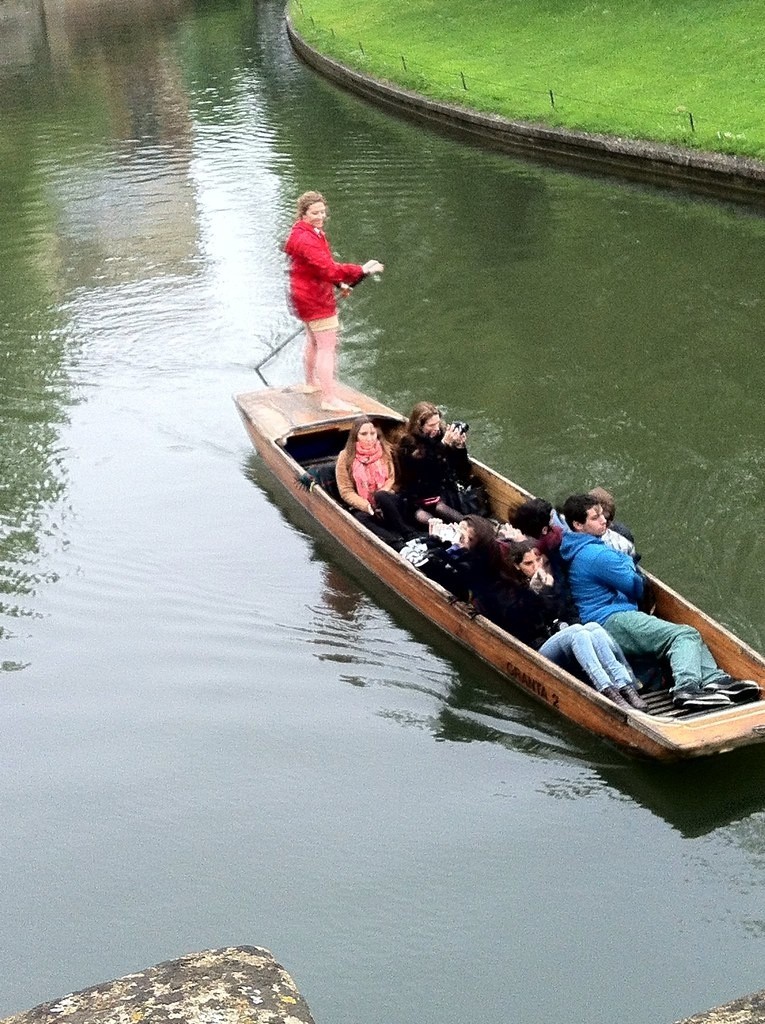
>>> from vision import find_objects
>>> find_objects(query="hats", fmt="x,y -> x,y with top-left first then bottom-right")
464,513 -> 495,543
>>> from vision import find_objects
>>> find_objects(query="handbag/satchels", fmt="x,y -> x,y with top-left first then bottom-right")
446,475 -> 492,518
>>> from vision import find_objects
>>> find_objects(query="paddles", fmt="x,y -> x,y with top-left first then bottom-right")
254,260 -> 381,372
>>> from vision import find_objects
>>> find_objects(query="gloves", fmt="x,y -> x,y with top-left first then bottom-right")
530,569 -> 554,593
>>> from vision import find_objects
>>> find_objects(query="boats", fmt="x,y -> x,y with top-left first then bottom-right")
233,375 -> 765,759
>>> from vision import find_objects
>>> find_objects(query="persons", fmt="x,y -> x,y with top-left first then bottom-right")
336,415 -> 436,529
282,190 -> 385,414
400,486 -> 761,710
396,401 -> 472,521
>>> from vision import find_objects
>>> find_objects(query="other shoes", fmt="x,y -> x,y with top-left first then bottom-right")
619,683 -> 647,711
672,690 -> 731,707
703,676 -> 759,695
602,686 -> 633,710
399,537 -> 429,567
